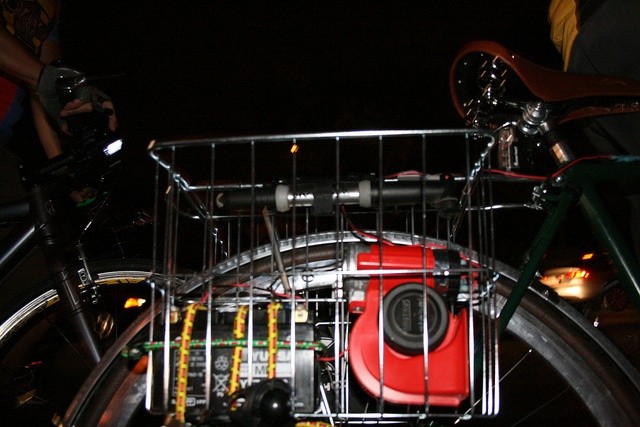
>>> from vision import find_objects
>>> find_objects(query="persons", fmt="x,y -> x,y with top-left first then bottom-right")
1,0 -> 118,203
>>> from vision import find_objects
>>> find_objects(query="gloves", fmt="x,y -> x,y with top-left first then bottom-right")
35,63 -> 113,128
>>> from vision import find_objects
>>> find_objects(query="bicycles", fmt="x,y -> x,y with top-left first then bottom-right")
0,75 -> 186,427
59,43 -> 640,427
